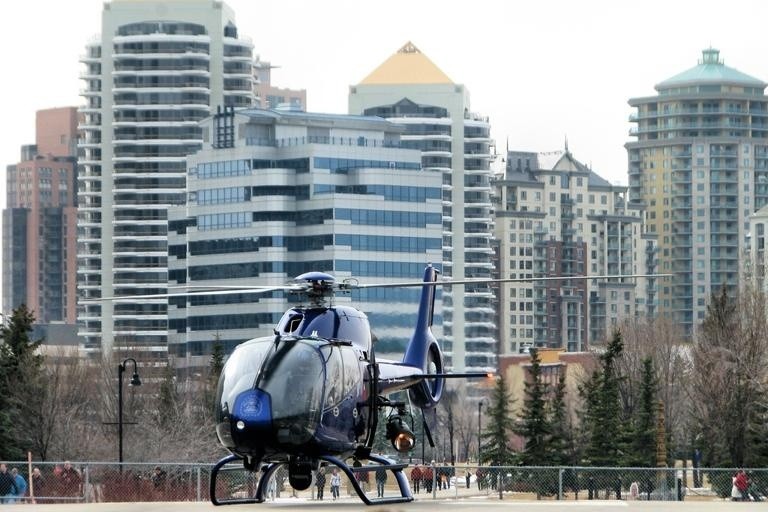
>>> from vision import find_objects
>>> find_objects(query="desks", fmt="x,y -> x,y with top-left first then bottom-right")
478,395 -> 491,465
118,358 -> 141,474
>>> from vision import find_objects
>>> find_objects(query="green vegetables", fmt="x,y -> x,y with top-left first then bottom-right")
82,264 -> 672,505
